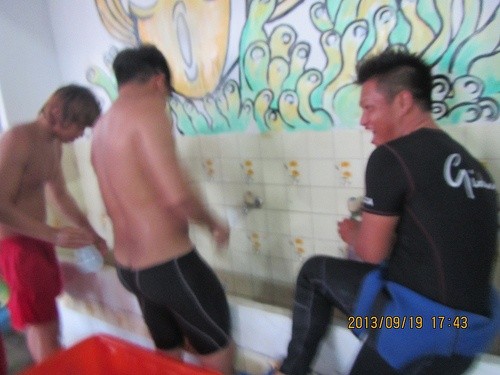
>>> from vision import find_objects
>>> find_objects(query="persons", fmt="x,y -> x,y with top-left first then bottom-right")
0,82 -> 108,363
260,52 -> 500,375
89,46 -> 240,375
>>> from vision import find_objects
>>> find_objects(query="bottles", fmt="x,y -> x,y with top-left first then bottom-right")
76,242 -> 104,272
